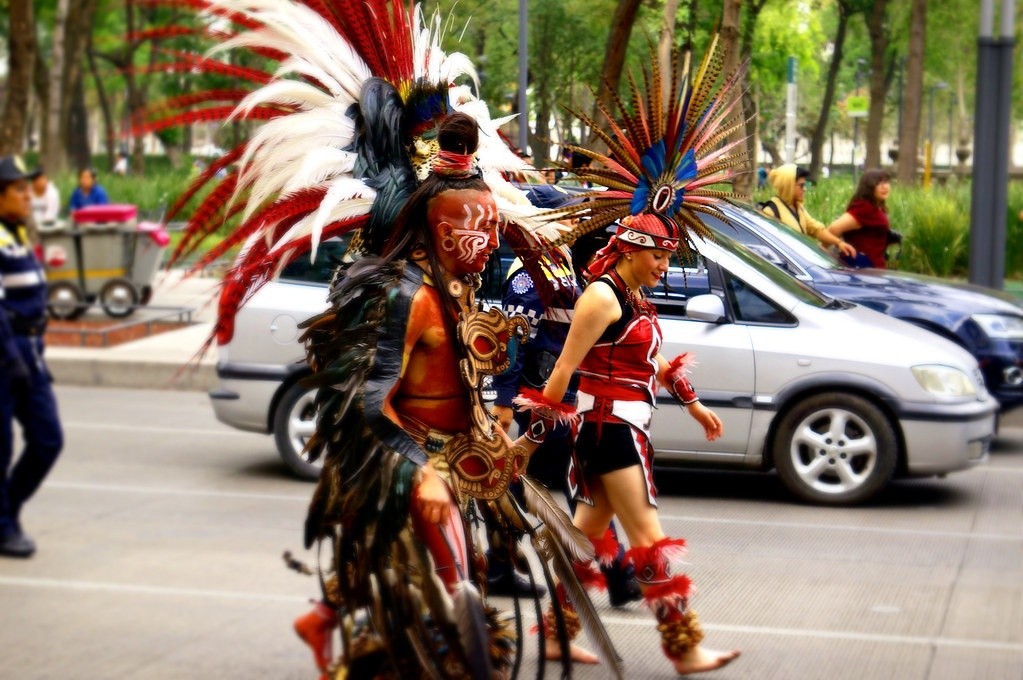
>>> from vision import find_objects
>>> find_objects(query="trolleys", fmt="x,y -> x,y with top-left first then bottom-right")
34,222 -> 167,320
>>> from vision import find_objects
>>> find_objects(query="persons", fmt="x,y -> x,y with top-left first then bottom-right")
28,167 -> 60,220
756,164 -> 892,270
0,159 -> 65,559
67,166 -> 108,216
112,153 -> 127,173
291,119 -> 741,680
194,157 -> 205,172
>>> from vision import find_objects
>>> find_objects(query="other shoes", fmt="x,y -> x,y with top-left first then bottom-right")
602,564 -> 643,605
0,524 -> 35,557
485,546 -> 547,597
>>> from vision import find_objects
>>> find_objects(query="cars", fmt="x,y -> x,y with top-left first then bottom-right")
207,183 -> 1023,507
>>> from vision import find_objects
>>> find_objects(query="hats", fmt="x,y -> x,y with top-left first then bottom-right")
0,156 -> 42,180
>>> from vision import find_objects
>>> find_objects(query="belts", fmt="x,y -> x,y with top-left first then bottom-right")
14,325 -> 44,339
